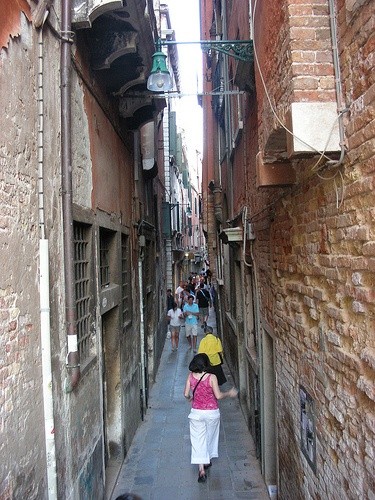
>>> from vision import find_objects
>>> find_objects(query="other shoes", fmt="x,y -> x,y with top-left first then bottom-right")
172,347 -> 174,351
176,348 -> 179,350
187,346 -> 192,351
193,349 -> 197,354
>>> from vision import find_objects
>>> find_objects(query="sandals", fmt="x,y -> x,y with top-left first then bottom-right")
198,470 -> 207,482
204,461 -> 212,469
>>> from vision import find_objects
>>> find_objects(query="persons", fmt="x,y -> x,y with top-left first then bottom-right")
197,326 -> 227,385
183,295 -> 199,353
167,302 -> 184,351
167,268 -> 216,328
184,353 -> 238,482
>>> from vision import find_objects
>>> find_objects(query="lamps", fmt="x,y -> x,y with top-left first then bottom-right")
146,37 -> 253,94
